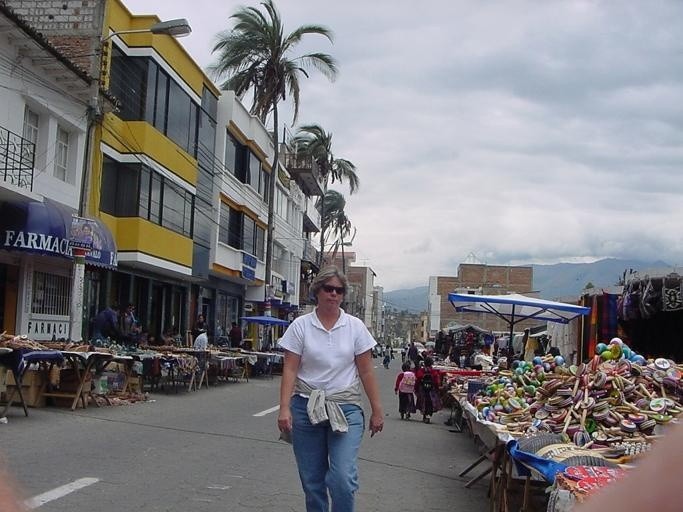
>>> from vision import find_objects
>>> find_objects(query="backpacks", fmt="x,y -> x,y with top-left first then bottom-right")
420,371 -> 434,391
399,372 -> 416,393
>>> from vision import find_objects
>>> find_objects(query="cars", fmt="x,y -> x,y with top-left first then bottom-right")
372,343 -> 385,358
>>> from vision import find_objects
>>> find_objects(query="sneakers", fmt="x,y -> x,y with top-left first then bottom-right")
423,418 -> 430,423
401,414 -> 410,420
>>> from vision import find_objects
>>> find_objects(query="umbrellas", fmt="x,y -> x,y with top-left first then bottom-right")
239,315 -> 290,347
448,292 -> 593,370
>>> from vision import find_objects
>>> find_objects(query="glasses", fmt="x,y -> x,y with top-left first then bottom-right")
321,285 -> 345,294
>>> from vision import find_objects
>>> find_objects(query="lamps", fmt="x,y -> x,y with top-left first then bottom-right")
102,16 -> 192,43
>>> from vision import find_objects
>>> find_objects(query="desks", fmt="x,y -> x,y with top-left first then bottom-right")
1,350 -> 276,422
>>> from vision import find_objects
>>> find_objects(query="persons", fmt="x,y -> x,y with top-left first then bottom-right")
190,315 -> 208,348
414,357 -> 441,424
94,303 -> 182,345
394,361 -> 417,420
79,224 -> 96,249
276,264 -> 385,512
228,322 -> 243,347
384,342 -> 419,368
